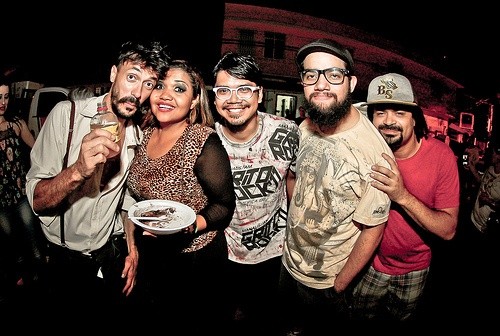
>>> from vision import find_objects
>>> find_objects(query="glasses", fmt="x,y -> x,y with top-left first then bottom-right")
213,86 -> 260,100
298,68 -> 349,85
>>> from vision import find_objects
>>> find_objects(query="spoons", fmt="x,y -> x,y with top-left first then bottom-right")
127,216 -> 172,222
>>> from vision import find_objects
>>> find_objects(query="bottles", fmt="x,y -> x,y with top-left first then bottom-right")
90,102 -> 121,158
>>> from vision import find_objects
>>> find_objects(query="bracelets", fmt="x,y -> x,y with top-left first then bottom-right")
182,227 -> 189,234
192,220 -> 198,235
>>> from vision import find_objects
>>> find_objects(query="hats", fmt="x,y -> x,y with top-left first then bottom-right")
360,73 -> 417,107
295,38 -> 354,76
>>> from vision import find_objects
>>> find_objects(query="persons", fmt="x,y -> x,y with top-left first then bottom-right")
0,71 -> 52,287
284,105 -> 307,127
276,39 -> 397,336
126,59 -> 237,336
346,72 -> 460,336
457,130 -> 500,234
208,51 -> 301,336
25,41 -> 173,336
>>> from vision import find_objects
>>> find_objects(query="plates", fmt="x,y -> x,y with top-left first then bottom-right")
128,199 -> 196,234
